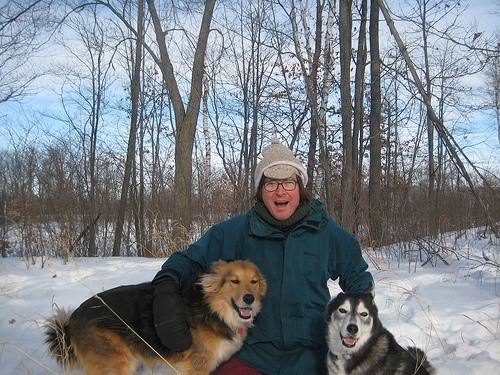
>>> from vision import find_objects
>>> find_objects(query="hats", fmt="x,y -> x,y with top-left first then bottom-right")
255,138 -> 308,194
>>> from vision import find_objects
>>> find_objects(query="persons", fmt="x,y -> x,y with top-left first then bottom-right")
151,136 -> 375,375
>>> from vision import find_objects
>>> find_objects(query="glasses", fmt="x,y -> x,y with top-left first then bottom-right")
261,179 -> 298,191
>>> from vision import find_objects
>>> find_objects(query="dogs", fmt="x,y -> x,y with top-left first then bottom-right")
40,259 -> 270,375
325,280 -> 438,375
